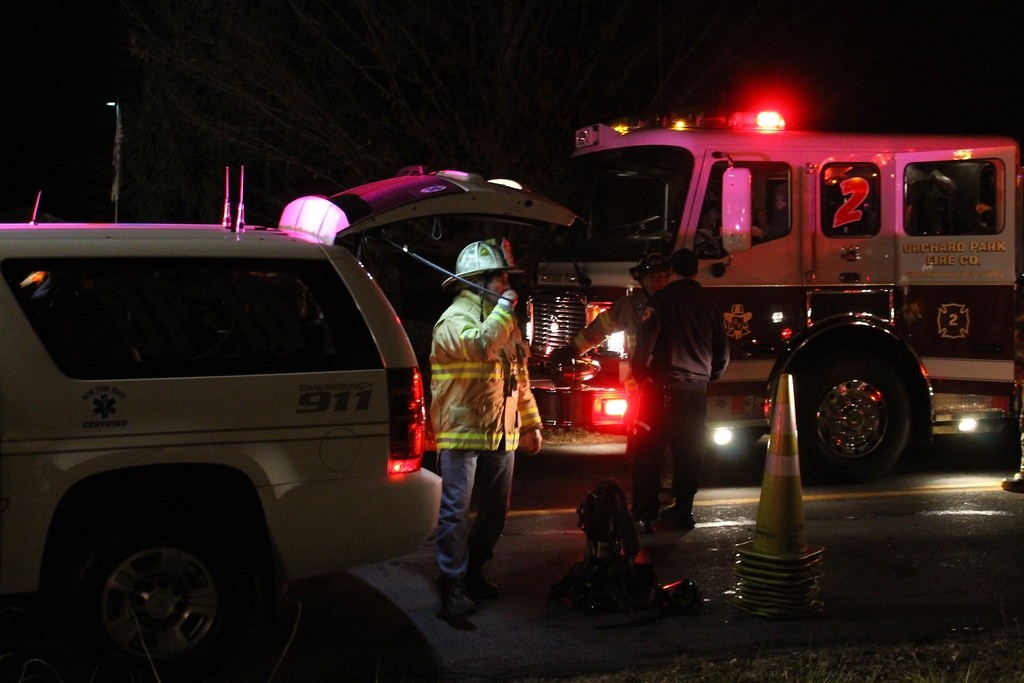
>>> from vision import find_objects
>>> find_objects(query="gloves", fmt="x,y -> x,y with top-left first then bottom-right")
559,342 -> 579,364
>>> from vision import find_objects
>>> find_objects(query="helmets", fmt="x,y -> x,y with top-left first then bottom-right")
442,241 -> 511,286
629,252 -> 672,270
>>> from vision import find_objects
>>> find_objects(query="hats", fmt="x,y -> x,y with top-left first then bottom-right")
669,248 -> 699,276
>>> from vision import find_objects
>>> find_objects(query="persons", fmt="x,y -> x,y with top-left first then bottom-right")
550,254 -> 676,506
430,242 -> 544,617
701,194 -> 765,246
631,249 -> 730,533
1002,272 -> 1024,492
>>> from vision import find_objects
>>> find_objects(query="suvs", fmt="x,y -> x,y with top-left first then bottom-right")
0,163 -> 588,683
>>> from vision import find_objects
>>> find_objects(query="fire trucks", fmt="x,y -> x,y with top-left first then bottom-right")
527,114 -> 1023,479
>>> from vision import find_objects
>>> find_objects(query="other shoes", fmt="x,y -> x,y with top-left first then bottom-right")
660,507 -> 695,529
460,570 -> 500,602
1002,474 -> 1024,493
434,578 -> 457,619
631,502 -> 659,520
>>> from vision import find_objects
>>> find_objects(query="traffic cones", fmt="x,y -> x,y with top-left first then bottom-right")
726,368 -> 827,625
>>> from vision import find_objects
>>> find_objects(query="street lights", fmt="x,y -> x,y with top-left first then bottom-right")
106,100 -> 127,225
